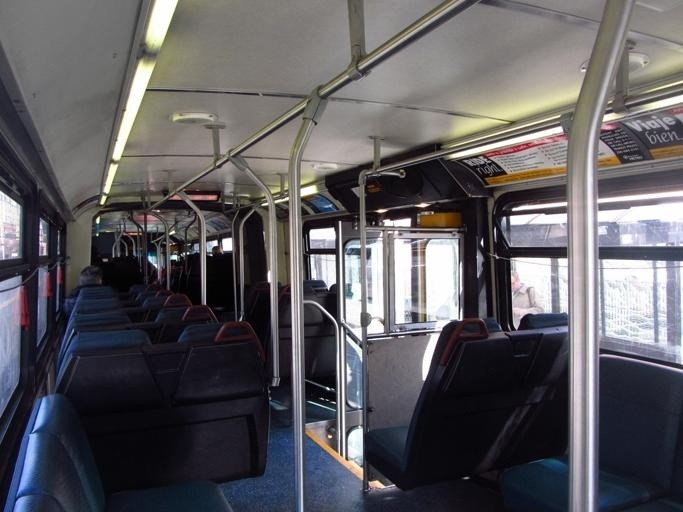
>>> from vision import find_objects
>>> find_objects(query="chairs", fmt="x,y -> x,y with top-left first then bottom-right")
163,249 -> 335,401
3,279 -> 275,512
363,311 -> 683,512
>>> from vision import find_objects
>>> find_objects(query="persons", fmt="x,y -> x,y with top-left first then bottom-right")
212,246 -> 224,257
508,271 -> 545,330
71,265 -> 102,296
